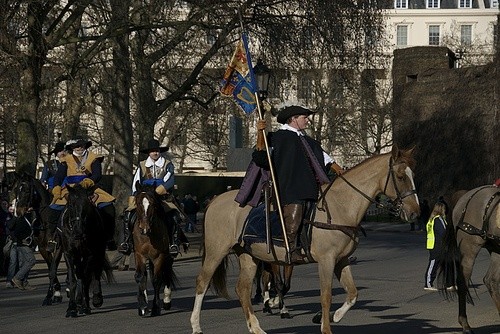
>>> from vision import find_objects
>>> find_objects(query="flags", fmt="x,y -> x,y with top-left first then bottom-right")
218,28 -> 258,114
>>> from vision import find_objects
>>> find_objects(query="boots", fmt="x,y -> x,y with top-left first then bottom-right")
45,209 -> 59,252
285,203 -> 304,264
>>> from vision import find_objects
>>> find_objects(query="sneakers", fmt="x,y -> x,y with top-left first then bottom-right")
5,279 -> 31,289
424,286 -> 438,291
447,285 -> 458,290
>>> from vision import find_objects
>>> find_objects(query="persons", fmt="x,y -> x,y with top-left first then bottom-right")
4,206 -> 24,289
119,140 -> 180,256
11,201 -> 38,291
46,134 -> 118,254
1,199 -> 10,257
423,201 -> 459,292
252,106 -> 346,264
40,142 -> 67,197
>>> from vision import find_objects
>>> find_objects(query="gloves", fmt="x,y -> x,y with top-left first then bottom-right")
80,177 -> 95,189
254,120 -> 269,150
331,162 -> 346,174
53,185 -> 62,201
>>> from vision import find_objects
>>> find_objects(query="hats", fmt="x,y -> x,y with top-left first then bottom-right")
275,106 -> 318,124
46,143 -> 65,154
139,139 -> 169,154
64,136 -> 93,151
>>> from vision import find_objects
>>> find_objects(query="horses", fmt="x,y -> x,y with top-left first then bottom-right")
12,170 -> 118,306
436,185 -> 500,333
128,180 -> 174,318
56,183 -> 108,318
189,142 -> 421,334
